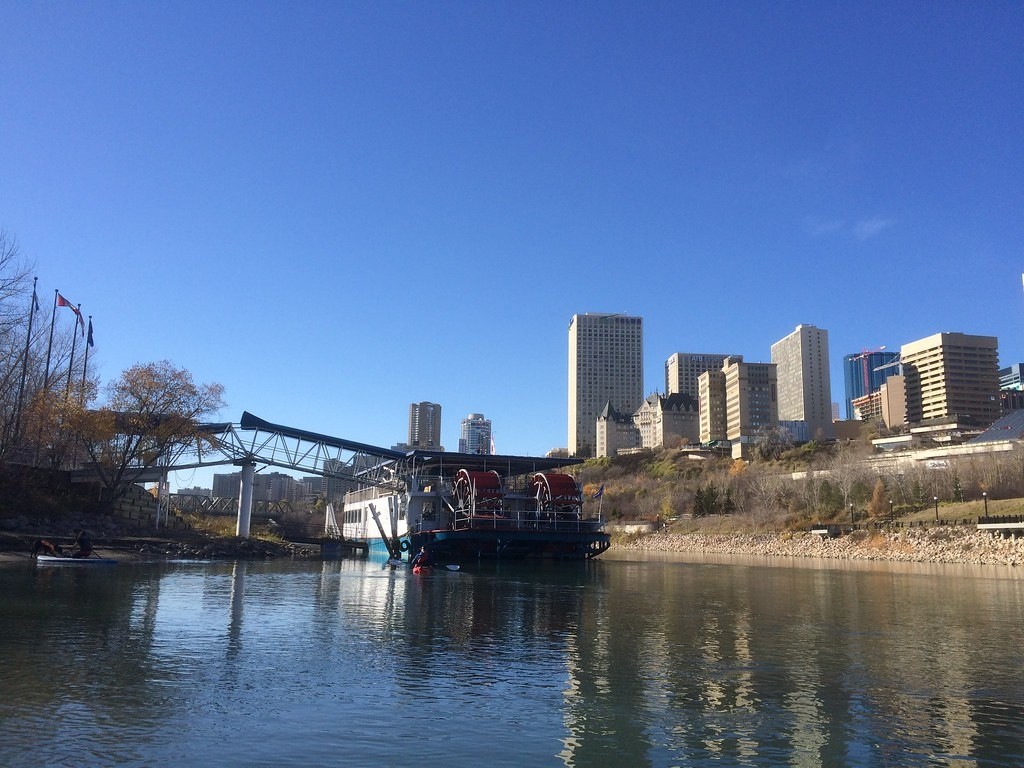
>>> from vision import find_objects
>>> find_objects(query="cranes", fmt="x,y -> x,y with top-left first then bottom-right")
848,345 -> 886,395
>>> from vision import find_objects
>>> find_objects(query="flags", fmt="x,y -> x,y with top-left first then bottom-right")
78,308 -> 86,337
56,293 -> 80,314
89,320 -> 96,347
33,282 -> 41,312
593,483 -> 604,498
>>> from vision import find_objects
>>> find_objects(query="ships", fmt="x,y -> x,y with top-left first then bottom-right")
325,447 -> 614,571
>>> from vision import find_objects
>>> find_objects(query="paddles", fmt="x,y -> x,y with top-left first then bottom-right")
91,550 -> 102,559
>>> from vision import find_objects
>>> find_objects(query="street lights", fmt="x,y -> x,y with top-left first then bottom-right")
933,496 -> 938,522
850,504 -> 854,524
982,492 -> 987,518
889,501 -> 893,522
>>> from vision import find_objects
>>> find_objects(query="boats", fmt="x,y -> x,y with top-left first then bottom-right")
37,554 -> 120,565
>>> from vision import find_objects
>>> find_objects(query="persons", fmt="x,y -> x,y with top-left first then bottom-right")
71,527 -> 93,559
411,545 -> 431,566
